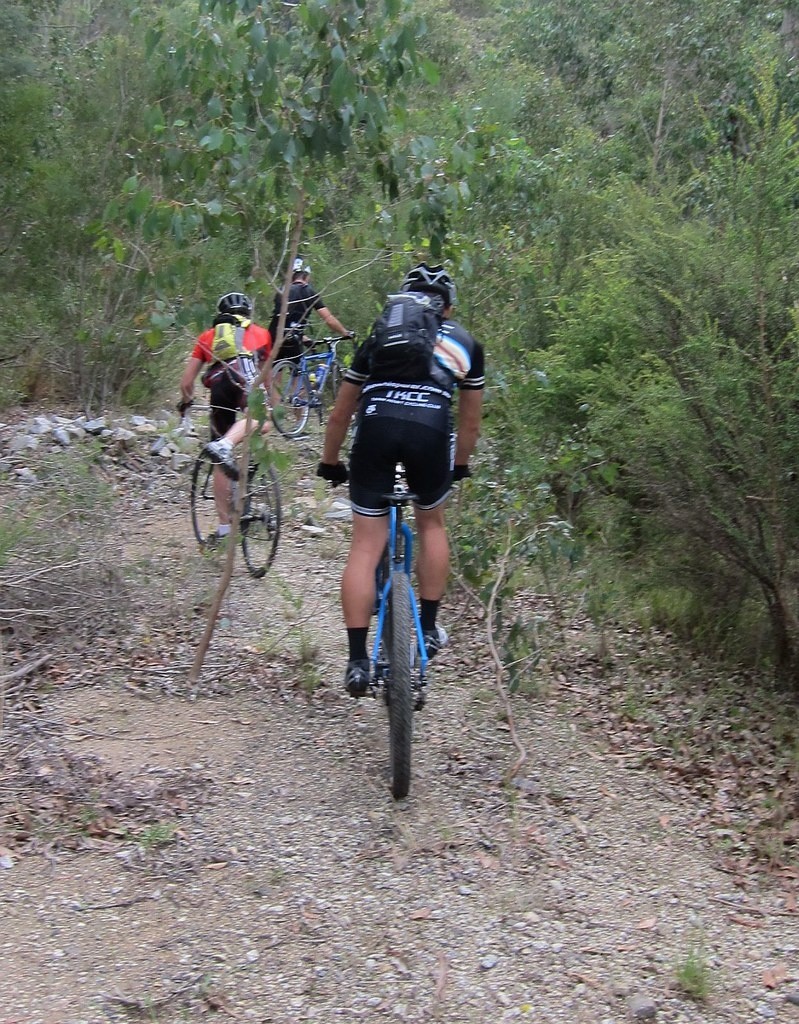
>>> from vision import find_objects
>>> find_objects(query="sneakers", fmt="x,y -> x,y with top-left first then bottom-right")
417,624 -> 448,660
203,440 -> 240,481
344,659 -> 370,699
207,530 -> 230,545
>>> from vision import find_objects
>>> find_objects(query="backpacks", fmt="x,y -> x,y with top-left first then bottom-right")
208,313 -> 253,368
359,291 -> 456,394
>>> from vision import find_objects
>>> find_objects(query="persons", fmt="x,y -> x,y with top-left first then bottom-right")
177,293 -> 279,547
322,263 -> 486,693
268,258 -> 357,442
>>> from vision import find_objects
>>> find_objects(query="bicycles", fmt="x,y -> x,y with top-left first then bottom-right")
269,329 -> 356,439
176,396 -> 284,579
315,468 -> 474,800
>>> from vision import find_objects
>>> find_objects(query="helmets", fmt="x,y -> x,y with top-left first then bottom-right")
215,291 -> 253,316
292,258 -> 311,275
399,261 -> 457,306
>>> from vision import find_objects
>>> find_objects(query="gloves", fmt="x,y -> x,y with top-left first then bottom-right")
453,464 -> 473,481
317,462 -> 347,488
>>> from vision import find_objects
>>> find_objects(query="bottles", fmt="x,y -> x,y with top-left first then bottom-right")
315,363 -> 326,385
308,371 -> 317,392
